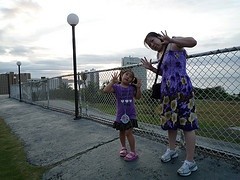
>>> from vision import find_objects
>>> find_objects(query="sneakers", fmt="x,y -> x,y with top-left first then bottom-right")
161,148 -> 179,163
176,159 -> 198,176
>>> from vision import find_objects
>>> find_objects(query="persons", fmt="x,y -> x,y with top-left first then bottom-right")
140,30 -> 198,176
104,69 -> 142,162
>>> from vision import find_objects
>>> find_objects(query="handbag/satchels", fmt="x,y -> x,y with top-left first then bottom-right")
152,83 -> 162,98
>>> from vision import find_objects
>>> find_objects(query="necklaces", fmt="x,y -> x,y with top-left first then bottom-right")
159,51 -> 163,55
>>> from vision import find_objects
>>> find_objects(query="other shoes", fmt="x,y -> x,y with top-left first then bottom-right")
120,148 -> 127,157
124,152 -> 138,161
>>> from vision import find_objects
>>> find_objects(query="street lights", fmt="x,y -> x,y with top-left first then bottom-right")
66,13 -> 81,120
16,61 -> 22,102
